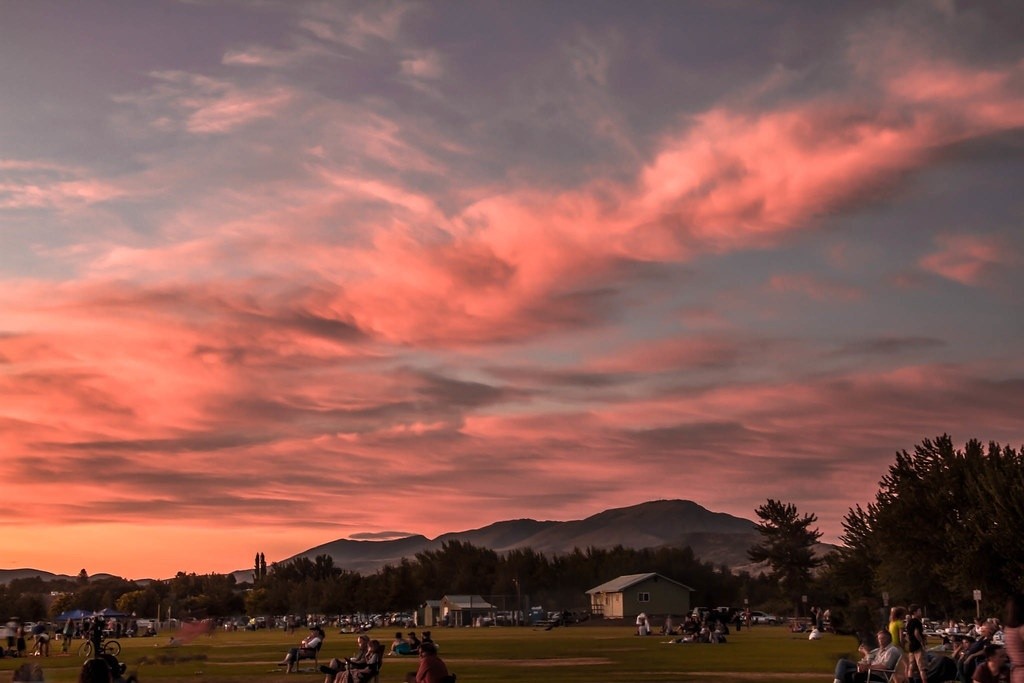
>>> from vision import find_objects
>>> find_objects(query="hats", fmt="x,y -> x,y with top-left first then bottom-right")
941,628 -> 960,637
309,626 -> 321,631
408,632 -> 416,635
421,631 -> 431,637
959,632 -> 976,642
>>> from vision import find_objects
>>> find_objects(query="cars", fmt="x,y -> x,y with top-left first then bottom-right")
256,611 -> 410,627
692,605 -> 780,625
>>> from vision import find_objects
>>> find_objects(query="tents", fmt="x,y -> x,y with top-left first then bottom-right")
55,607 -> 90,628
95,608 -> 127,617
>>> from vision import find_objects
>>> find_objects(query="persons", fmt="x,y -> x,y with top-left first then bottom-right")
890,605 -> 909,683
278,623 -> 325,673
0,609 -> 158,683
391,631 -> 440,656
662,605 -> 754,643
942,615 -> 1024,683
320,635 -> 370,683
809,626 -> 825,641
445,613 -> 450,628
635,612 -> 652,635
833,629 -> 899,683
334,640 -> 382,683
815,606 -> 824,632
908,604 -> 928,683
810,607 -> 817,626
223,613 -> 370,633
416,641 -> 450,683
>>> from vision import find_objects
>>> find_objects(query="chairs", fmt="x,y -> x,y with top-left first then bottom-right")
296,642 -> 323,672
867,654 -> 903,683
359,643 -> 386,683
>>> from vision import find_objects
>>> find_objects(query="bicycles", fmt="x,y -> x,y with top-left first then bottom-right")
78,632 -> 122,659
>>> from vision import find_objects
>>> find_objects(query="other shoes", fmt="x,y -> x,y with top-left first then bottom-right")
277,663 -> 287,666
320,665 -> 336,675
286,670 -> 296,674
